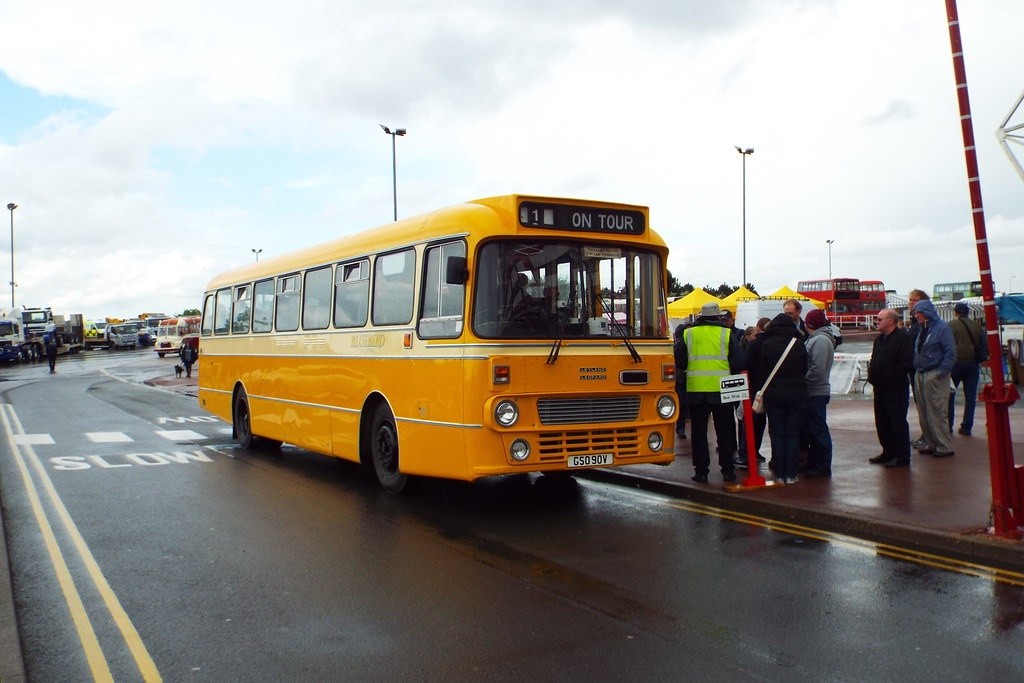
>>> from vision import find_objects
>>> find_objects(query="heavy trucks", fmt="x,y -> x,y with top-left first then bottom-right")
0,306 -> 171,366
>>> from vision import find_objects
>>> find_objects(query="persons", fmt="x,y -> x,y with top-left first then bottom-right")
671,299 -> 833,486
183,342 -> 196,377
491,265 -> 533,319
947,303 -> 988,435
45,318 -> 61,373
867,309 -> 914,467
896,314 -> 907,332
908,291 -> 931,448
914,299 -> 956,457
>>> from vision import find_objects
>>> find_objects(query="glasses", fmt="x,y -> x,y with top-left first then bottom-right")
878,318 -> 887,321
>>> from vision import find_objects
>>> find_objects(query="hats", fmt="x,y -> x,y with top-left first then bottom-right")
698,303 -> 728,316
954,302 -> 969,313
805,309 -> 826,330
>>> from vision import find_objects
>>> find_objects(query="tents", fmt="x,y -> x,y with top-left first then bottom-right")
667,285 -> 826,327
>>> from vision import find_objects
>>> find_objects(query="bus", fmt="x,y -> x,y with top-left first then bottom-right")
197,195 -> 680,497
153,315 -> 201,358
932,280 -> 996,301
797,278 -> 860,326
859,279 -> 886,327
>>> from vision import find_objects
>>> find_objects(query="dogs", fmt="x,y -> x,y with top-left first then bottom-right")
175,365 -> 185,377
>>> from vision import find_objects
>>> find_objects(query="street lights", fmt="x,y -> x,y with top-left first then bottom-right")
735,145 -> 754,287
378,123 -> 407,221
826,239 -> 834,279
7,202 -> 19,308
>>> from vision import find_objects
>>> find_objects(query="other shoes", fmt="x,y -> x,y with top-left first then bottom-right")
805,468 -> 832,477
933,451 -> 955,456
919,445 -> 935,454
950,377 -> 957,393
723,472 -> 737,481
869,453 -> 894,463
885,458 -> 910,467
959,427 -> 971,435
691,473 -> 708,483
775,477 -> 786,486
786,475 -> 800,484
911,435 -> 927,448
734,456 -> 748,468
677,432 -> 686,439
756,452 -> 766,461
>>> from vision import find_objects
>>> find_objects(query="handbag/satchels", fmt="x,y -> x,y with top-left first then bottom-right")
975,348 -> 987,362
752,391 -> 766,414
